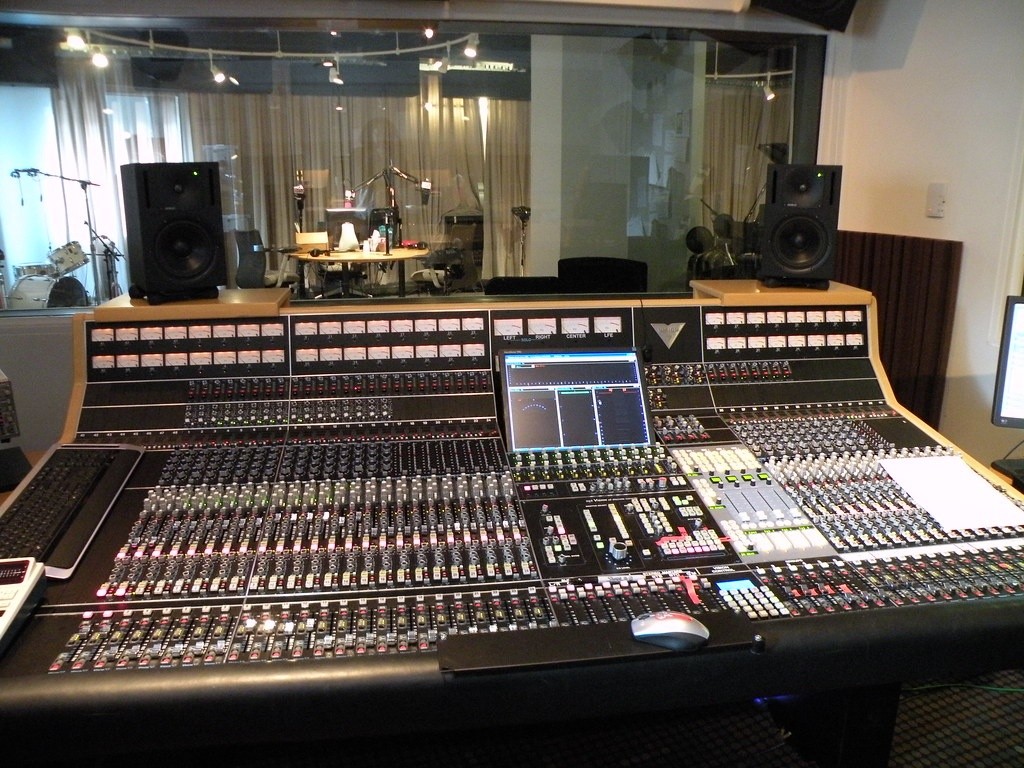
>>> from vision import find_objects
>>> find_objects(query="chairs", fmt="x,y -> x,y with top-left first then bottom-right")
315,208 -> 375,299
484,256 -> 647,295
234,229 -> 300,295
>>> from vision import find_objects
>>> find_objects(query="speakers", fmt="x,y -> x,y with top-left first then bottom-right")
120,161 -> 228,306
759,164 -> 843,290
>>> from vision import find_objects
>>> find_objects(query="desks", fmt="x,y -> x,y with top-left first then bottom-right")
285,245 -> 430,298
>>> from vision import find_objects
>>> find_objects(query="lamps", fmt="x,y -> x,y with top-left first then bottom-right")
90,46 -> 109,67
763,74 -> 775,100
328,67 -> 344,85
210,65 -> 225,83
463,33 -> 480,57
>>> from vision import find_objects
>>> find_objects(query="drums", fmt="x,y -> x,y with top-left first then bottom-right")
12,262 -> 54,281
46,239 -> 91,276
6,273 -> 89,310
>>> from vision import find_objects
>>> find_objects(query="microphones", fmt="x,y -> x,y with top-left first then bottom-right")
421,182 -> 431,205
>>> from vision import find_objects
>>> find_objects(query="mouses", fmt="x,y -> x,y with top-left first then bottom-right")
631,610 -> 709,650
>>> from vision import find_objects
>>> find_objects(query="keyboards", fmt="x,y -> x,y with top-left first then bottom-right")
0,444 -> 145,579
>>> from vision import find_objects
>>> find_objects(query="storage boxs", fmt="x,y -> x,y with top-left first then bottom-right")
445,215 -> 482,287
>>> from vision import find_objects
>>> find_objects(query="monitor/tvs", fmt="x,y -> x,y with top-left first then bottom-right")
991,296 -> 1024,428
326,209 -> 368,252
499,347 -> 656,453
